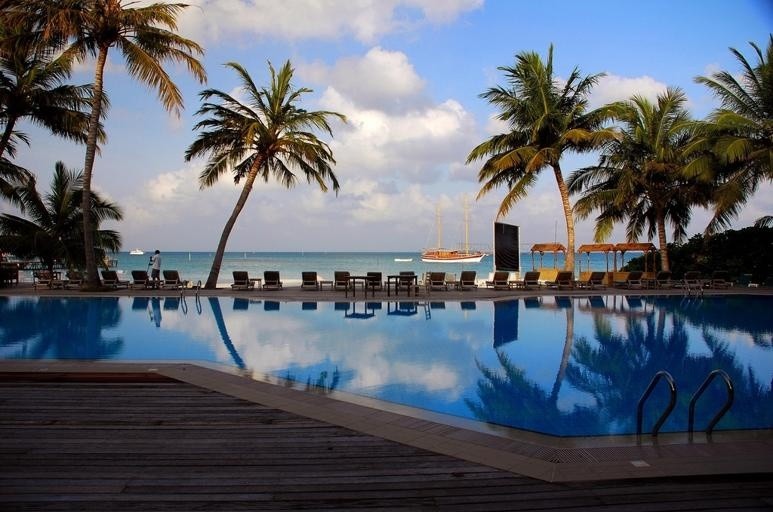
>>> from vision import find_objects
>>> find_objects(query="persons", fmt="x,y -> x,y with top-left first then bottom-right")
148,250 -> 161,289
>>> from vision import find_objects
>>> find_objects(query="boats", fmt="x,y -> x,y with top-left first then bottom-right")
393,257 -> 412,263
128,247 -> 143,256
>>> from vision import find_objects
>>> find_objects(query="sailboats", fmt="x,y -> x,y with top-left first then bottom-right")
418,190 -> 487,264
